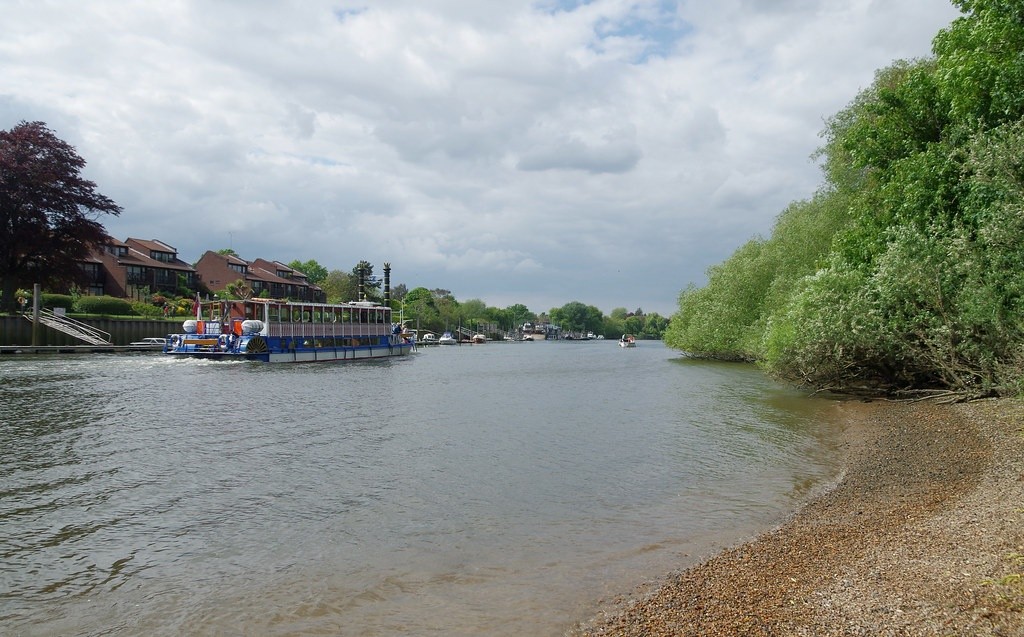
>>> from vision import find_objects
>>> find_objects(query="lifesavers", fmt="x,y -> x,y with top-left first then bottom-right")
170,334 -> 181,348
218,334 -> 228,350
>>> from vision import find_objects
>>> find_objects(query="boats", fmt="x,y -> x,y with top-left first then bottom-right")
162,289 -> 415,363
439,334 -> 457,345
473,334 -> 486,344
618,334 -> 636,348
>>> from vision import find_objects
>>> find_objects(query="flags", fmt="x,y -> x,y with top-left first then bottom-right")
192,295 -> 199,317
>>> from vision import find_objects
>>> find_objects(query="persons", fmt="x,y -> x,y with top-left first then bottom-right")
163,301 -> 179,320
622,333 -> 626,342
392,322 -> 409,345
